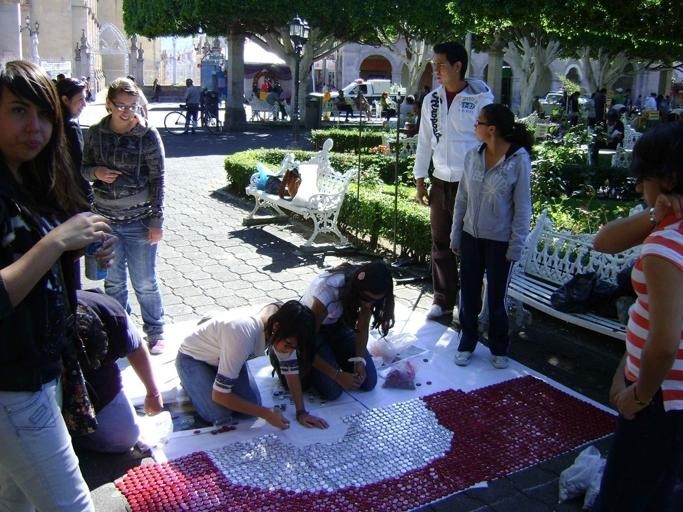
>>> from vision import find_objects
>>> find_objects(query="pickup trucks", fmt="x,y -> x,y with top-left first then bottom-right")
331,76 -> 405,108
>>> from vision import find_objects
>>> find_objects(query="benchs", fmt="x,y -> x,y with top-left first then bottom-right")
243,139 -> 356,253
250,98 -> 288,121
327,98 -> 373,121
477,204 -> 644,343
373,97 -> 398,120
610,125 -> 643,167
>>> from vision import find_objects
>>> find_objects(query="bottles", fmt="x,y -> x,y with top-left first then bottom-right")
85,234 -> 109,283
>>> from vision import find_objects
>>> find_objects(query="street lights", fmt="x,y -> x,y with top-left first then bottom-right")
288,14 -> 310,123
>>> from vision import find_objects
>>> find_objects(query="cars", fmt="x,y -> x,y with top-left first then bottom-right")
535,92 -> 590,119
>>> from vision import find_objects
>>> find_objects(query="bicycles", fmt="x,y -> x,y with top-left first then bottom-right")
164,101 -> 223,135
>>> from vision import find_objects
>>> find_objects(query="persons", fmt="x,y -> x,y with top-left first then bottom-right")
53,78 -> 95,204
531,88 -> 676,167
266,257 -> 395,403
0,60 -> 118,512
449,105 -> 534,369
175,299 -> 331,430
75,289 -> 163,454
81,76 -> 92,102
184,78 -> 201,134
57,74 -> 65,82
593,119 -> 682,512
334,89 -> 398,120
411,42 -> 495,322
128,75 -> 148,120
80,78 -> 164,353
250,76 -> 283,98
151,79 -> 161,102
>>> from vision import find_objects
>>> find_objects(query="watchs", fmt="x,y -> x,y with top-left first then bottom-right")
347,357 -> 368,368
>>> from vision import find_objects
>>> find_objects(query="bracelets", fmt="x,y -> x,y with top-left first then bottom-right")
332,369 -> 345,383
295,409 -> 310,415
146,393 -> 160,398
632,384 -> 652,410
648,206 -> 662,228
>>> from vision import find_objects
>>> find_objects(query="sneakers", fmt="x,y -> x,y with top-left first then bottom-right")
426,303 -> 453,319
477,311 -> 488,324
144,336 -> 164,355
454,351 -> 472,366
490,355 -> 509,368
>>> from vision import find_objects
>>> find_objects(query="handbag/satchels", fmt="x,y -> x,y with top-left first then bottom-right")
63,316 -> 97,438
549,269 -> 618,321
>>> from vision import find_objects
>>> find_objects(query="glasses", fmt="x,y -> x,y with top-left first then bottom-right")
281,343 -> 298,350
432,62 -> 449,69
473,120 -> 487,127
362,292 -> 382,306
111,99 -> 138,112
